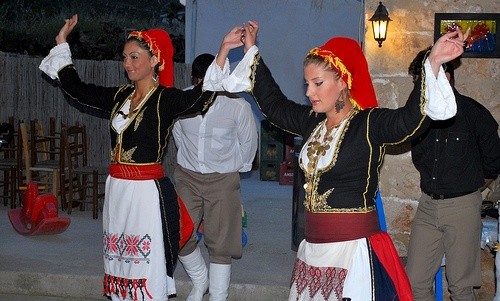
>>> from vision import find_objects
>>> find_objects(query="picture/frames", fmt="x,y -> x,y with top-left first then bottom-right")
434,13 -> 500,58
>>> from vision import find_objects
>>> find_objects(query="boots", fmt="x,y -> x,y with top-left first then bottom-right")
208,262 -> 232,301
178,245 -> 209,301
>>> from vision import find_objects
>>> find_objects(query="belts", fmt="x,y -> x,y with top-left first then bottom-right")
421,188 -> 479,200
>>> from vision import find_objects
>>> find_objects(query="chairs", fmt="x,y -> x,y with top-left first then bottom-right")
0,116 -> 108,219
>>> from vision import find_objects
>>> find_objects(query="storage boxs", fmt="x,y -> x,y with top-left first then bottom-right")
261,120 -> 294,184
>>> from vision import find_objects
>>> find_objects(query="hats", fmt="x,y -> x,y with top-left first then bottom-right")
191,53 -> 215,79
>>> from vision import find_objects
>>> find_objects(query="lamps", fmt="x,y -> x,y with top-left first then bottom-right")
369,2 -> 391,47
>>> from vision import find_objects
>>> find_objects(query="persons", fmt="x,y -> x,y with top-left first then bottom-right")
171,53 -> 258,301
38,14 -> 257,301
222,21 -> 471,301
405,45 -> 500,301
